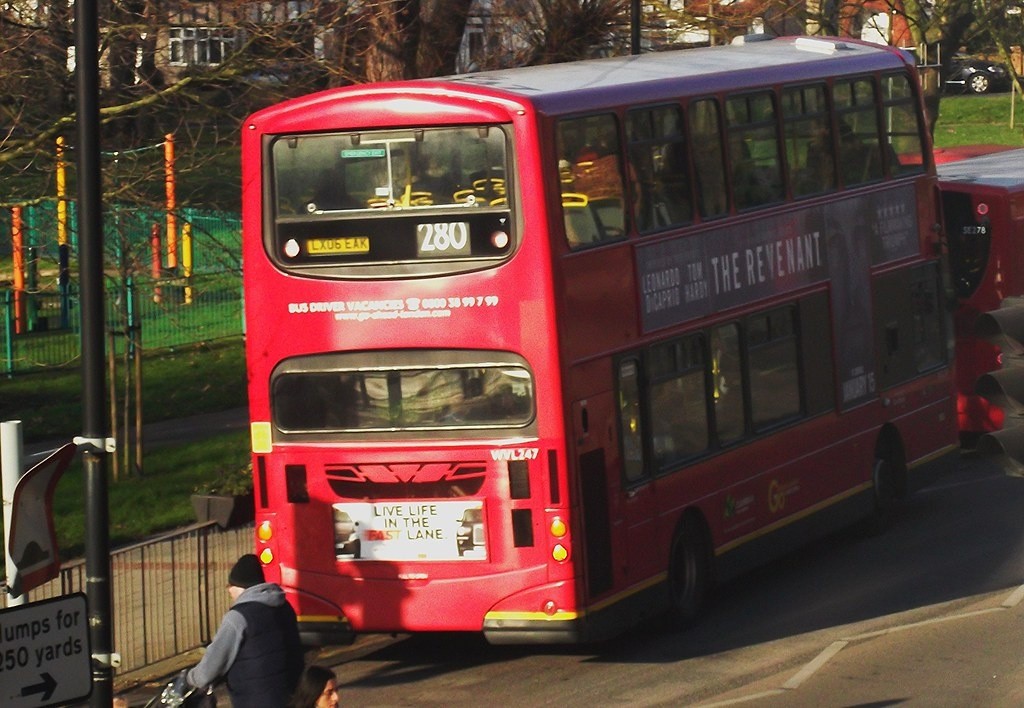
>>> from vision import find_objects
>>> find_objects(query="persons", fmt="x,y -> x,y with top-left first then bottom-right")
290,665 -> 340,708
661,114 -> 886,210
187,554 -> 304,708
447,378 -> 509,424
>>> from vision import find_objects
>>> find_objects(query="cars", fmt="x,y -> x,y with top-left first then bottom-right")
945,57 -> 1009,95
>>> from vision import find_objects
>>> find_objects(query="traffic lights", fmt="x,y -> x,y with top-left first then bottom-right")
975,297 -> 1024,478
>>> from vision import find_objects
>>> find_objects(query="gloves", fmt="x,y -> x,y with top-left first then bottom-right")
174,678 -> 192,697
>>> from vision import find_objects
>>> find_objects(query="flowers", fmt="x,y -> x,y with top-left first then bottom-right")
193,458 -> 255,497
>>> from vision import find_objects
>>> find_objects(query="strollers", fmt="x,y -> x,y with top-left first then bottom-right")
142,663 -> 215,708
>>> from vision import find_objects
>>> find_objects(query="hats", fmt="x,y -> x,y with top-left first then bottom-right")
228,554 -> 265,590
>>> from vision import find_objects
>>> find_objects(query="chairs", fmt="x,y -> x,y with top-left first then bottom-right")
365,161 -> 625,246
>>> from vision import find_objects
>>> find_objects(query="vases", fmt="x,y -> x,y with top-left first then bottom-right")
191,493 -> 254,532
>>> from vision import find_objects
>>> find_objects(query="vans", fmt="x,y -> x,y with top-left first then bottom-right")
900,140 -> 1024,436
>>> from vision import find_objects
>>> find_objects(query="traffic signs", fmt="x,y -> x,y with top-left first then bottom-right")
0,592 -> 94,708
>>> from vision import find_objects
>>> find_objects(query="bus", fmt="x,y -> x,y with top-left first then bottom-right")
242,32 -> 964,647
